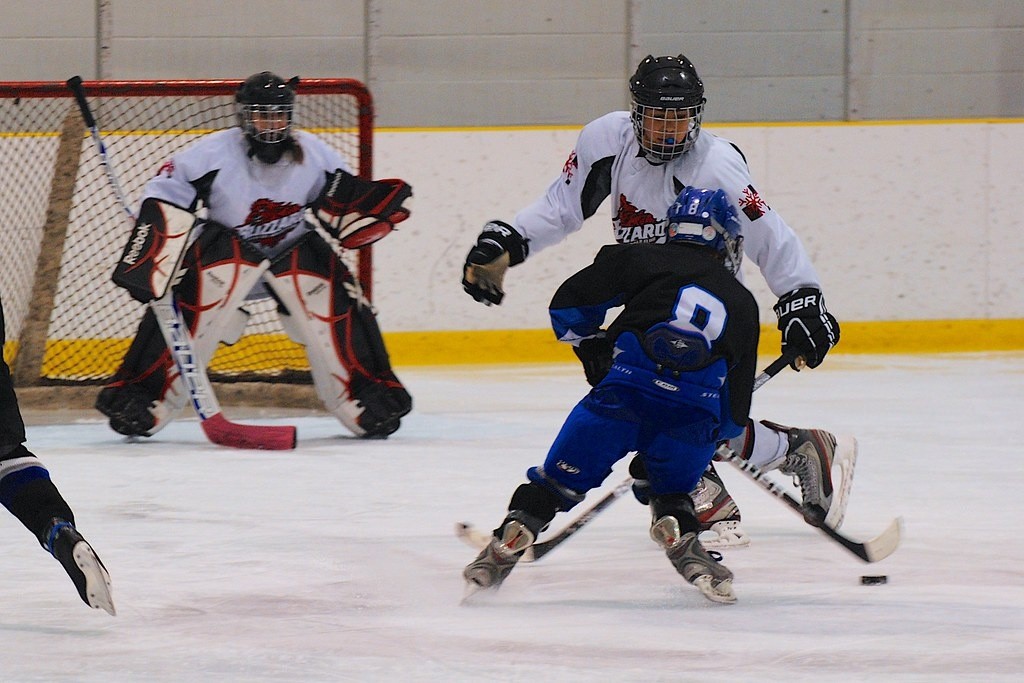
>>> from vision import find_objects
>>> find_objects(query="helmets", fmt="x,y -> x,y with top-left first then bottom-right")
234,71 -> 295,164
629,53 -> 707,163
665,185 -> 743,252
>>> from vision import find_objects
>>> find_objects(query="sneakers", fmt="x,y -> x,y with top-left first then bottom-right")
42,517 -> 116,616
456,511 -> 535,604
759,419 -> 858,534
650,515 -> 738,604
687,460 -> 751,550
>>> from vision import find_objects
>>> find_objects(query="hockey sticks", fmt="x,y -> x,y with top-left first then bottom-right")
66,76 -> 297,451
454,355 -> 790,564
712,443 -> 902,563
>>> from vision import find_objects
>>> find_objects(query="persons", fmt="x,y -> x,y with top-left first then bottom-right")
451,53 -> 858,606
94,72 -> 413,443
0,297 -> 115,615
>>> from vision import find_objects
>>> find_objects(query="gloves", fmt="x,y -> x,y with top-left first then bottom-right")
462,220 -> 530,306
572,329 -> 615,388
773,287 -> 840,372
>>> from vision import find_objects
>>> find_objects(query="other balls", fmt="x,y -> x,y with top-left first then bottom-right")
861,575 -> 887,585
